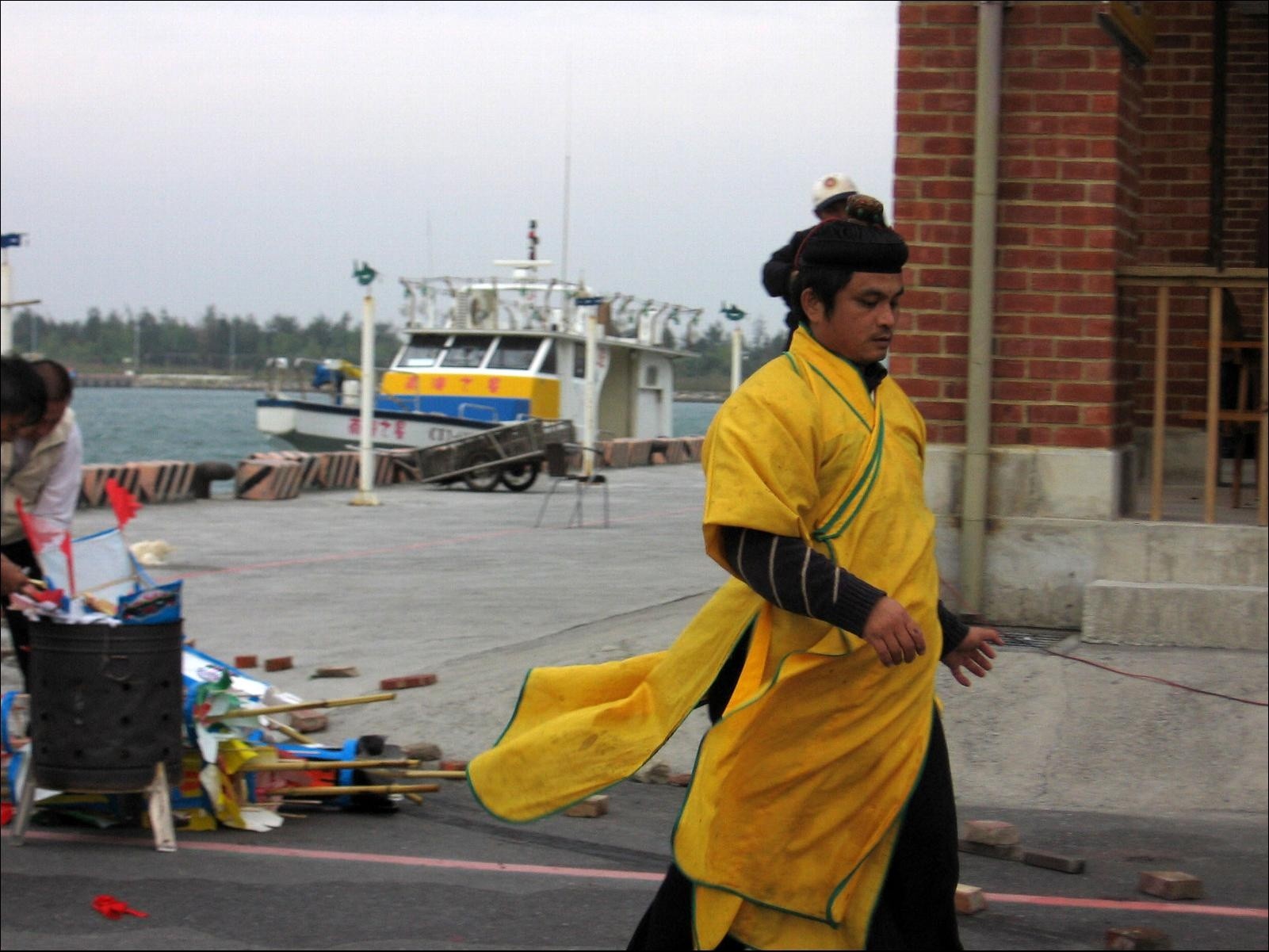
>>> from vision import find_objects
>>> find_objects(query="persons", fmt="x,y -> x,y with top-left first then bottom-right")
1,354 -> 49,445
465,173 -> 1006,951
0,356 -> 85,698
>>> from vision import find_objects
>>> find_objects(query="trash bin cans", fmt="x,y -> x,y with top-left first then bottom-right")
3,605 -> 183,854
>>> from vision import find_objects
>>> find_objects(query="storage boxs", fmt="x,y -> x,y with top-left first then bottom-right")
45,526 -> 184,628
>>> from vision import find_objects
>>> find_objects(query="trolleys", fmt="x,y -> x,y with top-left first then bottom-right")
349,417 -> 554,495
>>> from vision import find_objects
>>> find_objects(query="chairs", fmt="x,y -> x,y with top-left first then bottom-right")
1209,288 -> 1261,478
535,440 -> 613,528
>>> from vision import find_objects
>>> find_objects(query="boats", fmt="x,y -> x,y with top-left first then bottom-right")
250,150 -> 701,463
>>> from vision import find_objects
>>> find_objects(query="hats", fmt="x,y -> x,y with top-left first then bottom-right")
811,172 -> 855,209
